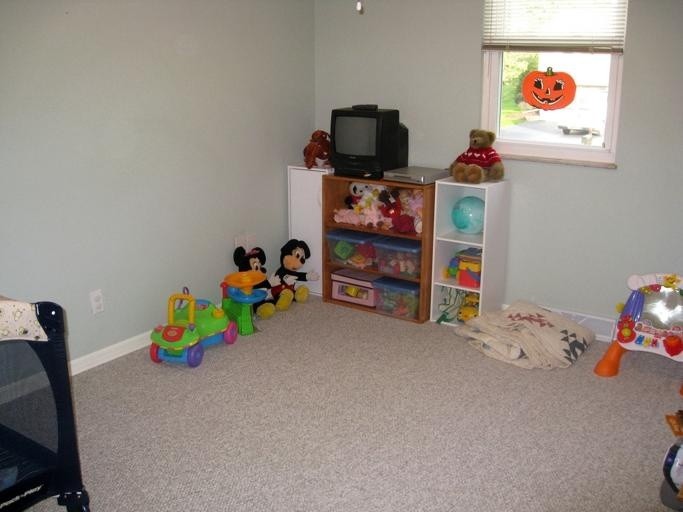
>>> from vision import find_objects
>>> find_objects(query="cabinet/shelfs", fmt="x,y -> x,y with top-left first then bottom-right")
318,161 -> 453,323
283,163 -> 335,300
426,176 -> 513,332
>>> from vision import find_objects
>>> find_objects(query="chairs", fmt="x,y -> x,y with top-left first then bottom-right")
1,296 -> 90,511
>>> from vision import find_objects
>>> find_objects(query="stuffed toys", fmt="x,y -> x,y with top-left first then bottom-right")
328,181 -> 424,319
233,246 -> 292,319
268,239 -> 321,303
449,129 -> 505,184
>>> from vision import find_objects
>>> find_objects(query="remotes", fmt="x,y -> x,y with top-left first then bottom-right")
352,105 -> 378,110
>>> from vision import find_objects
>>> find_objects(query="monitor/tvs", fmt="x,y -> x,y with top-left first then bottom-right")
331,108 -> 408,180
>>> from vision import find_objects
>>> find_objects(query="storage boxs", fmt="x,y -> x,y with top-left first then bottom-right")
369,276 -> 420,320
371,236 -> 422,279
323,228 -> 376,273
329,270 -> 377,310
